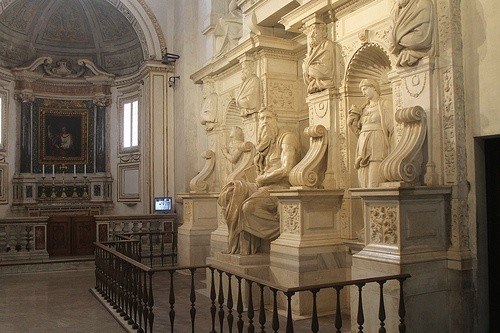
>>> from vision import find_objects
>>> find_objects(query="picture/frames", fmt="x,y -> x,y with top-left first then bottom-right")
39,106 -> 88,164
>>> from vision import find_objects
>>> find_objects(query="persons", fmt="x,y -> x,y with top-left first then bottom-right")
217,106 -> 302,256
347,79 -> 394,187
198,81 -> 218,126
301,22 -> 336,93
235,60 -> 261,115
220,127 -> 244,173
389,0 -> 435,67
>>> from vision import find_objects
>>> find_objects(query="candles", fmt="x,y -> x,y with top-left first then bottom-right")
52,163 -> 55,177
74,163 -> 76,176
43,164 -> 45,177
83,163 -> 86,176
